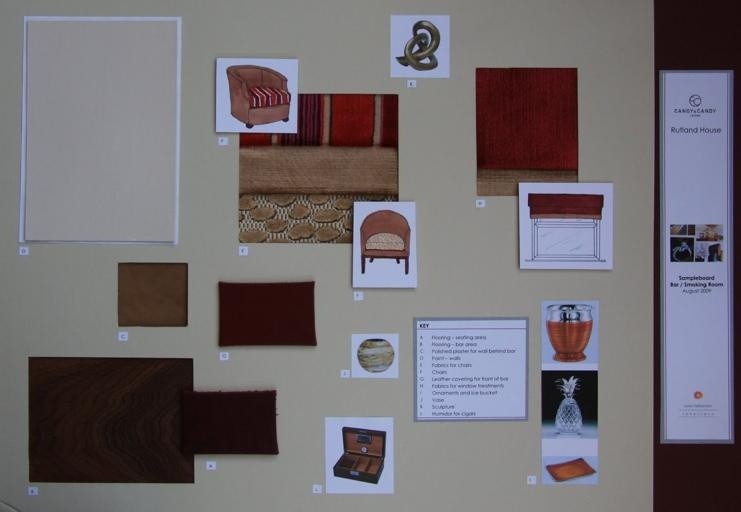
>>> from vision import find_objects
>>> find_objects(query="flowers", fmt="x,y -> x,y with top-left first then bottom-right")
228,64 -> 289,129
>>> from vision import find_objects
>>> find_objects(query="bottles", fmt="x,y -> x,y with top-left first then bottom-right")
555,393 -> 583,435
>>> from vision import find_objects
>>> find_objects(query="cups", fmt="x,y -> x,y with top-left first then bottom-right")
545,303 -> 594,362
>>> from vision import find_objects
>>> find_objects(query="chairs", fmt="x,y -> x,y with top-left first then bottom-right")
559,376 -> 579,397
361,209 -> 411,273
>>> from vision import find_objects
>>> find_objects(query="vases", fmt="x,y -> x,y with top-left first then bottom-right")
555,399 -> 583,434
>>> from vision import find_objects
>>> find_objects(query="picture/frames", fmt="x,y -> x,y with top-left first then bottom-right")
412,315 -> 530,423
658,67 -> 736,446
517,182 -> 614,269
